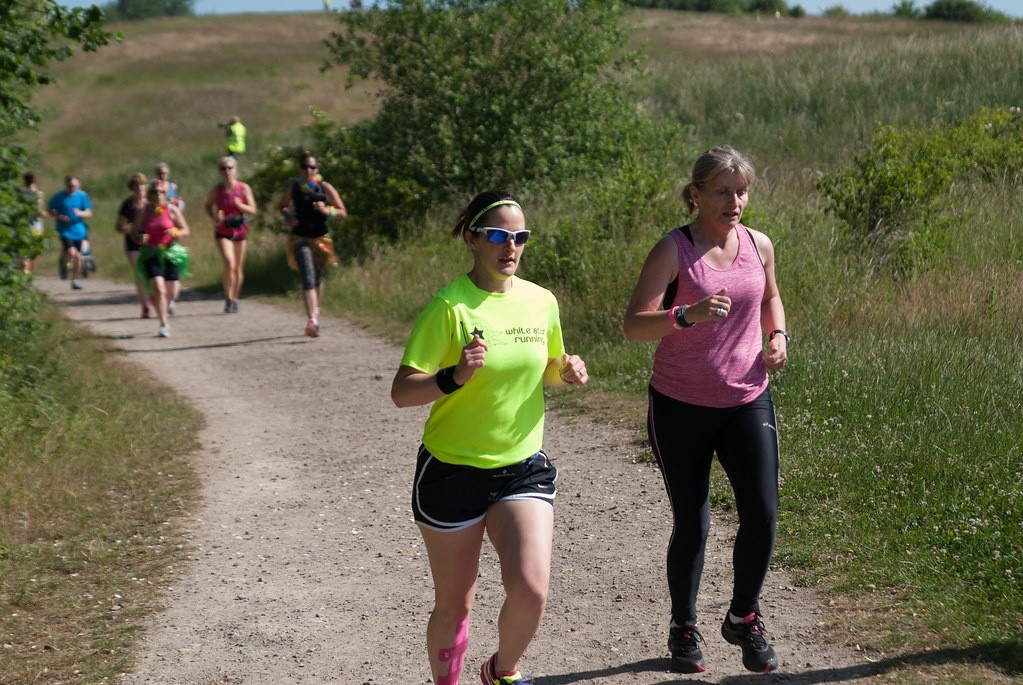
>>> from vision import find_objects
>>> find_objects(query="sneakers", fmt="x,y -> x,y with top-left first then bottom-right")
225,299 -> 238,313
668,623 -> 708,672
305,320 -> 319,337
480,652 -> 533,685
721,609 -> 778,673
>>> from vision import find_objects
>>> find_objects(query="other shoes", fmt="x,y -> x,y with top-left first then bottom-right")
168,300 -> 177,315
71,279 -> 82,290
157,323 -> 169,337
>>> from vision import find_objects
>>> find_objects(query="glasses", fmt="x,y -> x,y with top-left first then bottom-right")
300,163 -> 317,170
469,226 -> 531,245
219,165 -> 233,171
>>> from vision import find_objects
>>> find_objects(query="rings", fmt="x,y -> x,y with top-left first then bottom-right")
579,370 -> 583,376
716,307 -> 724,316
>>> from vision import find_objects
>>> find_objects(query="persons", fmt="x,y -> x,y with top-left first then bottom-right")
16,171 -> 48,274
129,179 -> 191,336
281,152 -> 346,337
625,146 -> 789,673
391,192 -> 588,685
225,115 -> 247,156
115,173 -> 156,317
48,174 -> 93,289
204,155 -> 257,314
149,162 -> 185,211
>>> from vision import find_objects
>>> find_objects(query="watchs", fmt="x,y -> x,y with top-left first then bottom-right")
676,306 -> 691,327
768,330 -> 790,342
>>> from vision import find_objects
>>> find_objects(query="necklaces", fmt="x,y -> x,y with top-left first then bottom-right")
296,174 -> 322,192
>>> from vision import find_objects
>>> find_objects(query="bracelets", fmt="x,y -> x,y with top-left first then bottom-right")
328,206 -> 336,221
436,365 -> 464,394
172,226 -> 179,240
669,305 -> 682,329
558,365 -> 573,385
